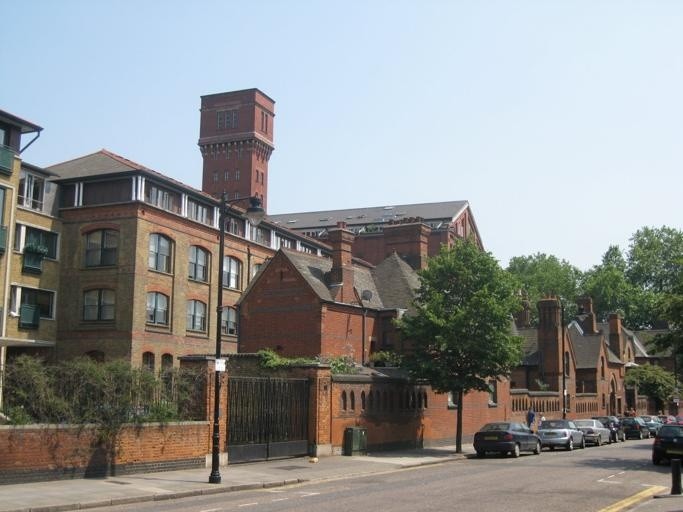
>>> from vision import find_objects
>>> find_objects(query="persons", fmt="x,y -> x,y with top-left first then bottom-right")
526,407 -> 535,428
624,407 -> 629,417
629,407 -> 636,416
538,411 -> 545,430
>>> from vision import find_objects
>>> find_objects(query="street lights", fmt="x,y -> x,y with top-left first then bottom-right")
208,187 -> 267,485
561,302 -> 589,420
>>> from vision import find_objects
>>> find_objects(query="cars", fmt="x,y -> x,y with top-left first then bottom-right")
472,414 -> 683,466
